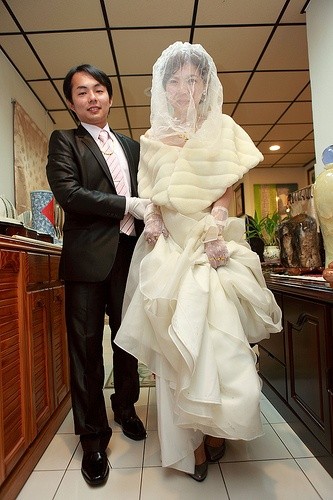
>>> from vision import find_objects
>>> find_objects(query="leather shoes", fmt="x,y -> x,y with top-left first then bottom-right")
111,412 -> 146,441
81,450 -> 109,485
204,435 -> 226,462
188,441 -> 208,482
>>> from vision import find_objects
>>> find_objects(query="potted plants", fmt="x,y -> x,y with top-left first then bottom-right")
244,210 -> 290,264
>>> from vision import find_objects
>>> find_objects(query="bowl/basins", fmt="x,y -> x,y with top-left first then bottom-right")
0,196 -> 19,222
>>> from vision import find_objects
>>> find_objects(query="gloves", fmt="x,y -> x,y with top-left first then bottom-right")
128,197 -> 152,220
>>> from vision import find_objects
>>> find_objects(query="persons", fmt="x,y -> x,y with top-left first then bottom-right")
46,65 -> 152,486
114,40 -> 282,481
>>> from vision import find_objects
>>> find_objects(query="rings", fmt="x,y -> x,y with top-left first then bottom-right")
210,257 -> 224,262
147,236 -> 159,242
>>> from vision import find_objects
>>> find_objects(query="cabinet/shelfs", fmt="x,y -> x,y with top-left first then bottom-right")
249,274 -> 333,479
0,236 -> 72,500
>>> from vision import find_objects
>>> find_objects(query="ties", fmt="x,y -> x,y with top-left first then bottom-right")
98,131 -> 135,236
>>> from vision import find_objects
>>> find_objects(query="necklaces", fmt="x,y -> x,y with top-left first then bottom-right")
101,151 -> 113,156
167,116 -> 203,142
